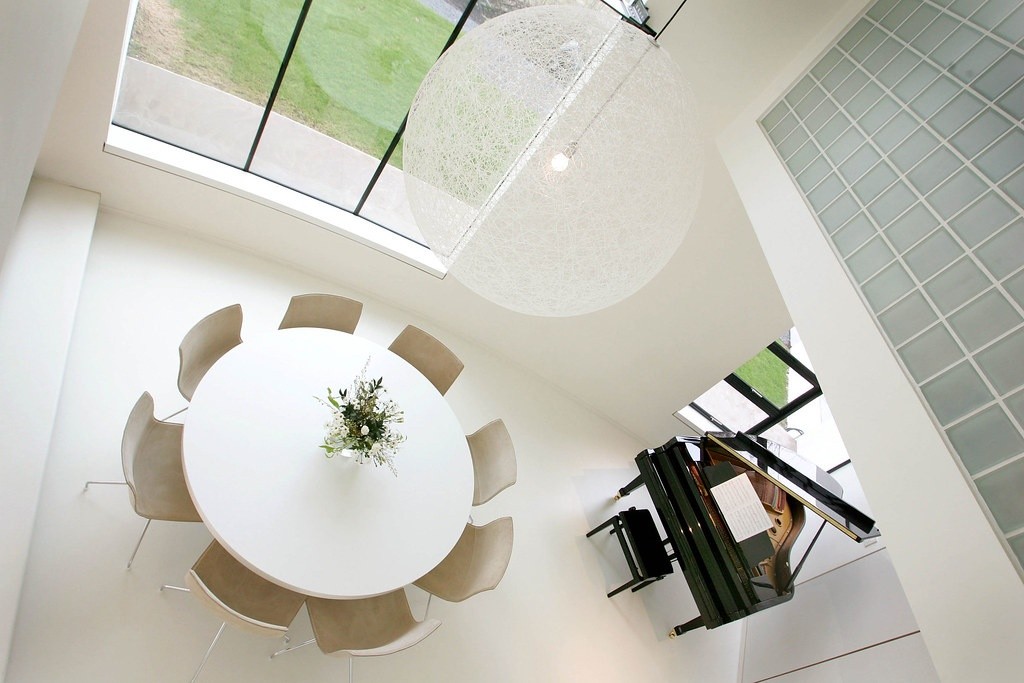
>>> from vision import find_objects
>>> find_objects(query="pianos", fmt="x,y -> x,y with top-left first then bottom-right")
614,430 -> 882,642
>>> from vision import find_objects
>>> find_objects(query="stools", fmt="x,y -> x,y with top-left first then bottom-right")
586,506 -> 674,598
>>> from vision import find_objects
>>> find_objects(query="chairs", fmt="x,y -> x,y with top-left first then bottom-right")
81,295 -> 516,683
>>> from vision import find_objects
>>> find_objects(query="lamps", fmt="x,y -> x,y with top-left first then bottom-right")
401,0 -> 705,318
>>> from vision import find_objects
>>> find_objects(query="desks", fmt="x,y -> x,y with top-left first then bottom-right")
182,327 -> 475,600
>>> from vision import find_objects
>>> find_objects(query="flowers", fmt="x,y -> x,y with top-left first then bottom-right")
311,353 -> 408,477
